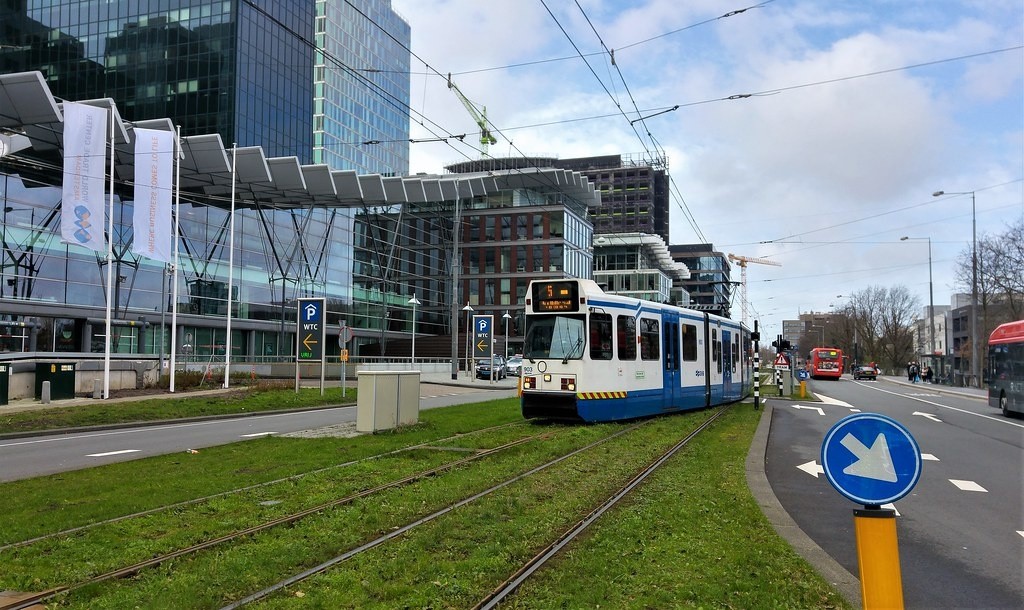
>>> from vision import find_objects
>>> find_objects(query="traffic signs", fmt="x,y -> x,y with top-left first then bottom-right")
472,315 -> 494,359
296,298 -> 326,361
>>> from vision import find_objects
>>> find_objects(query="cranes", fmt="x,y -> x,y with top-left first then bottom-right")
727,252 -> 783,325
447,72 -> 497,160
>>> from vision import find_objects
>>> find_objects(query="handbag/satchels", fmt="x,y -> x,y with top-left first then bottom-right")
915,374 -> 919,382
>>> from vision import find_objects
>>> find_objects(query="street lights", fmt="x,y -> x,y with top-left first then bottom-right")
461,301 -> 475,377
900,236 -> 935,353
407,292 -> 422,371
837,294 -> 857,365
502,309 -> 511,358
809,324 -> 825,348
932,190 -> 981,386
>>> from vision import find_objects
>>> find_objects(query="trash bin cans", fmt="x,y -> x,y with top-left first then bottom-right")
34,362 -> 77,400
955,373 -> 963,387
0,363 -> 11,405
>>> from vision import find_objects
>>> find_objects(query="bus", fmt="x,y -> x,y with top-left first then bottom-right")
986,319 -> 1024,417
808,347 -> 843,380
516,276 -> 755,422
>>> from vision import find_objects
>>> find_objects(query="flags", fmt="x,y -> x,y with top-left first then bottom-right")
60,101 -> 107,251
131,127 -> 174,265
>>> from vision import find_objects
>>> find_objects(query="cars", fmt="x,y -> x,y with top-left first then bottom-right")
476,354 -> 523,381
853,367 -> 877,381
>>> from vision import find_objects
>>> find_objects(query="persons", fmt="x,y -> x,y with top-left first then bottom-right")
874,364 -> 880,371
921,366 -> 928,384
927,366 -> 932,384
842,364 -> 845,373
907,361 -> 920,383
870,362 -> 875,368
851,362 -> 855,375
